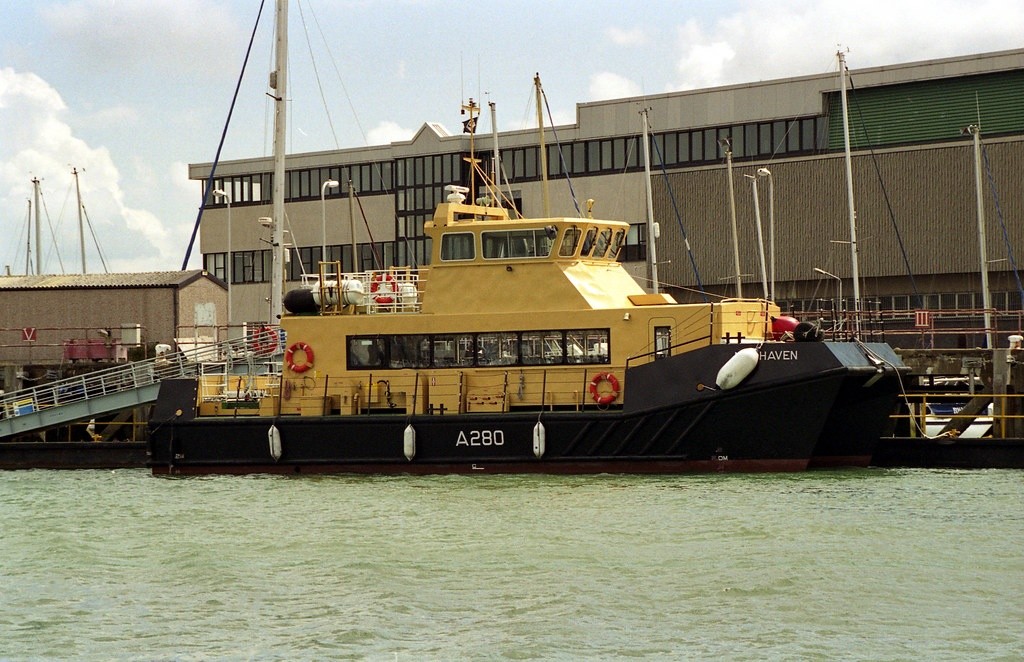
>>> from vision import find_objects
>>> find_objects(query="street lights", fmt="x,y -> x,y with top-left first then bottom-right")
812,267 -> 842,343
757,169 -> 775,303
212,189 -> 231,340
717,138 -> 742,302
321,180 -> 339,280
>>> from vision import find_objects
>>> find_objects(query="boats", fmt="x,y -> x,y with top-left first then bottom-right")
145,73 -> 913,476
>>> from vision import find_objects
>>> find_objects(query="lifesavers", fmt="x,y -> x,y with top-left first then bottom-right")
252,328 -> 279,352
372,273 -> 399,303
286,341 -> 315,373
590,372 -> 620,406
